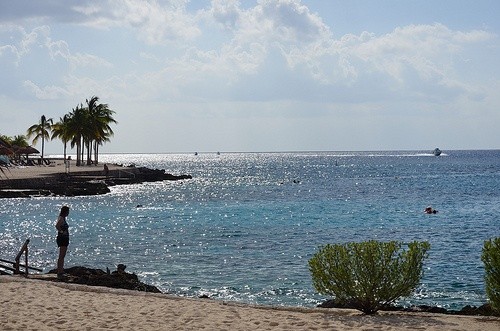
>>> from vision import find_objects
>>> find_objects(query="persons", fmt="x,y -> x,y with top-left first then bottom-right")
424,206 -> 432,213
55,206 -> 71,278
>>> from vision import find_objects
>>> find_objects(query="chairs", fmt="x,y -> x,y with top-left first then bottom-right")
9,156 -> 56,166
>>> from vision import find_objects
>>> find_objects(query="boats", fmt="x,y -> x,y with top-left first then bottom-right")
433,148 -> 441,156
